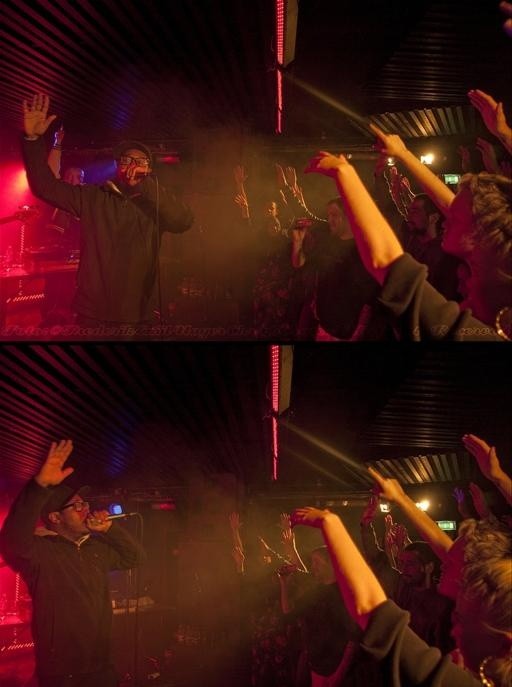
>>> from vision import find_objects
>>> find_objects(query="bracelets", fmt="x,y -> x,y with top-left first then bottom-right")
53,142 -> 63,152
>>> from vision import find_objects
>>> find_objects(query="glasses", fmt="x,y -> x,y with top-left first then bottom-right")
58,502 -> 89,513
115,155 -> 150,168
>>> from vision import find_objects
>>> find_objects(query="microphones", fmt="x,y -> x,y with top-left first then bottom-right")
132,168 -> 148,182
85,509 -> 138,526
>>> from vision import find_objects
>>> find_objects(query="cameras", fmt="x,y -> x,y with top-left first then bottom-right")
442,171 -> 461,188
433,518 -> 458,534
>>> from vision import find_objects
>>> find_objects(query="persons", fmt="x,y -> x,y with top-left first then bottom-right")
19,84 -> 196,345
0,437 -> 150,686
41,124 -> 85,244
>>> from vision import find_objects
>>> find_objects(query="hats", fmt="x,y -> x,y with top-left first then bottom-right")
111,141 -> 153,168
38,484 -> 90,524
